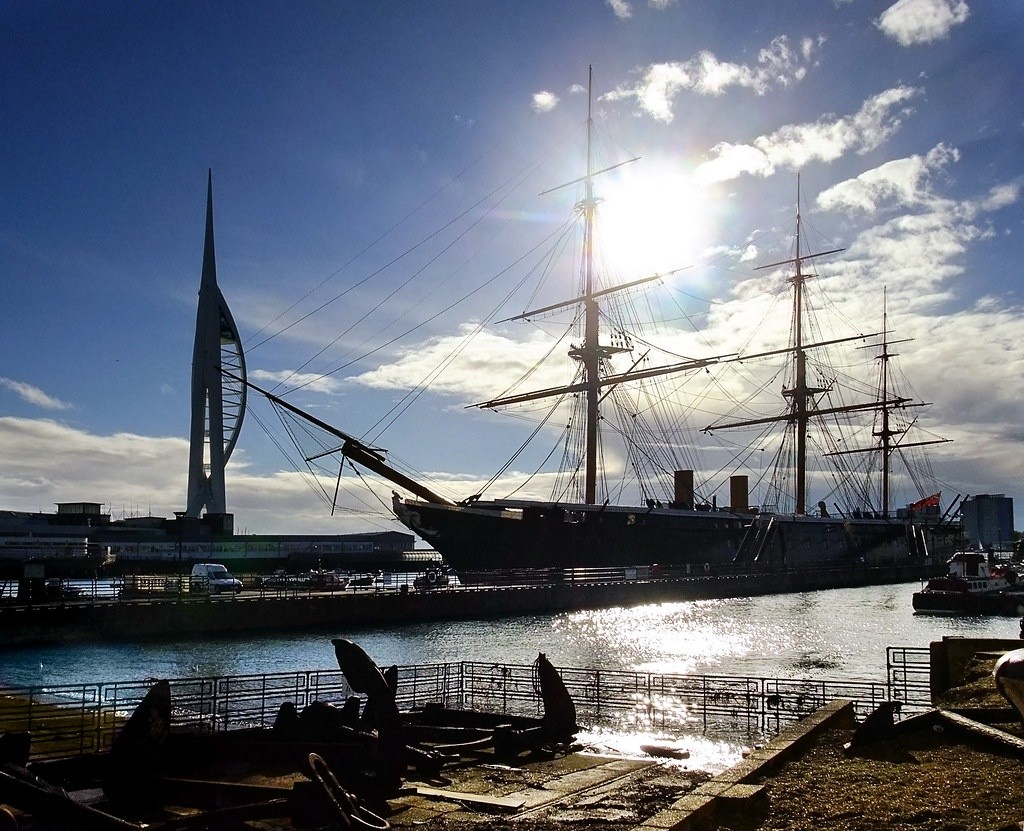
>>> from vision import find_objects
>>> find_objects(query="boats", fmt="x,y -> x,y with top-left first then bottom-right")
352,577 -> 373,585
306,573 -> 348,589
913,514 -> 1024,617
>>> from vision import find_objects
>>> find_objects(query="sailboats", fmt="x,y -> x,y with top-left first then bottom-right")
214,63 -> 972,589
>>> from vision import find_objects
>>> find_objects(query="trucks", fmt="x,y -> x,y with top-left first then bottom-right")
191,564 -> 243,595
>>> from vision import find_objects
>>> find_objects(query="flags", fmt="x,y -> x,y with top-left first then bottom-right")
912,492 -> 940,513
